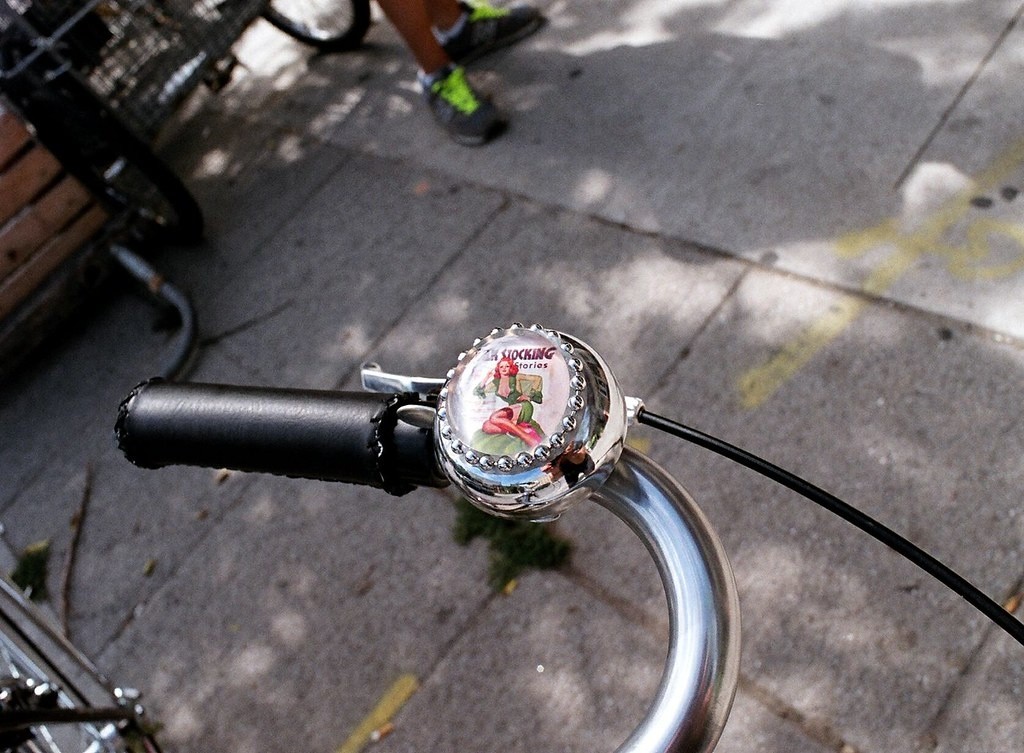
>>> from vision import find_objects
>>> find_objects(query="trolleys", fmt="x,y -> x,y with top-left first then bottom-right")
0,0 -> 373,259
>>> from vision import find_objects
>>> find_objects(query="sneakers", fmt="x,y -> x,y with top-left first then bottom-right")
416,65 -> 508,147
440,1 -> 547,66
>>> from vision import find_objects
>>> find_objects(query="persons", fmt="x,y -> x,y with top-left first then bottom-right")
376,0 -> 543,146
541,439 -> 595,490
472,356 -> 547,449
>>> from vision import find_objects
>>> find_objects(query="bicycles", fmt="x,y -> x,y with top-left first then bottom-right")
0,367 -> 1023,753
